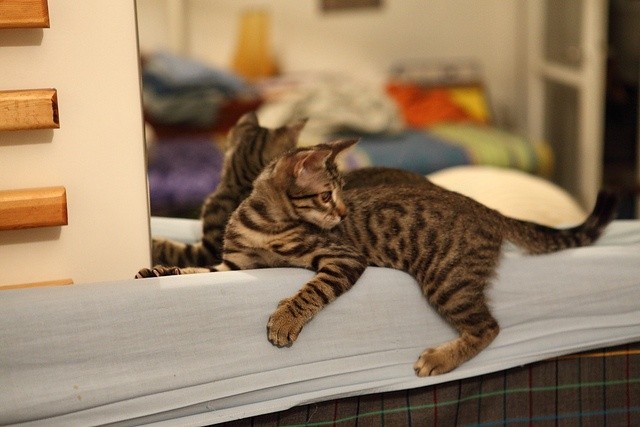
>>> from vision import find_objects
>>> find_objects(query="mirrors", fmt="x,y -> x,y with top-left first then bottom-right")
132,1 -> 639,269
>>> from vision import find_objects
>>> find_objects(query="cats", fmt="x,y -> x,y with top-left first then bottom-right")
134,137 -> 620,377
152,110 -> 437,268
